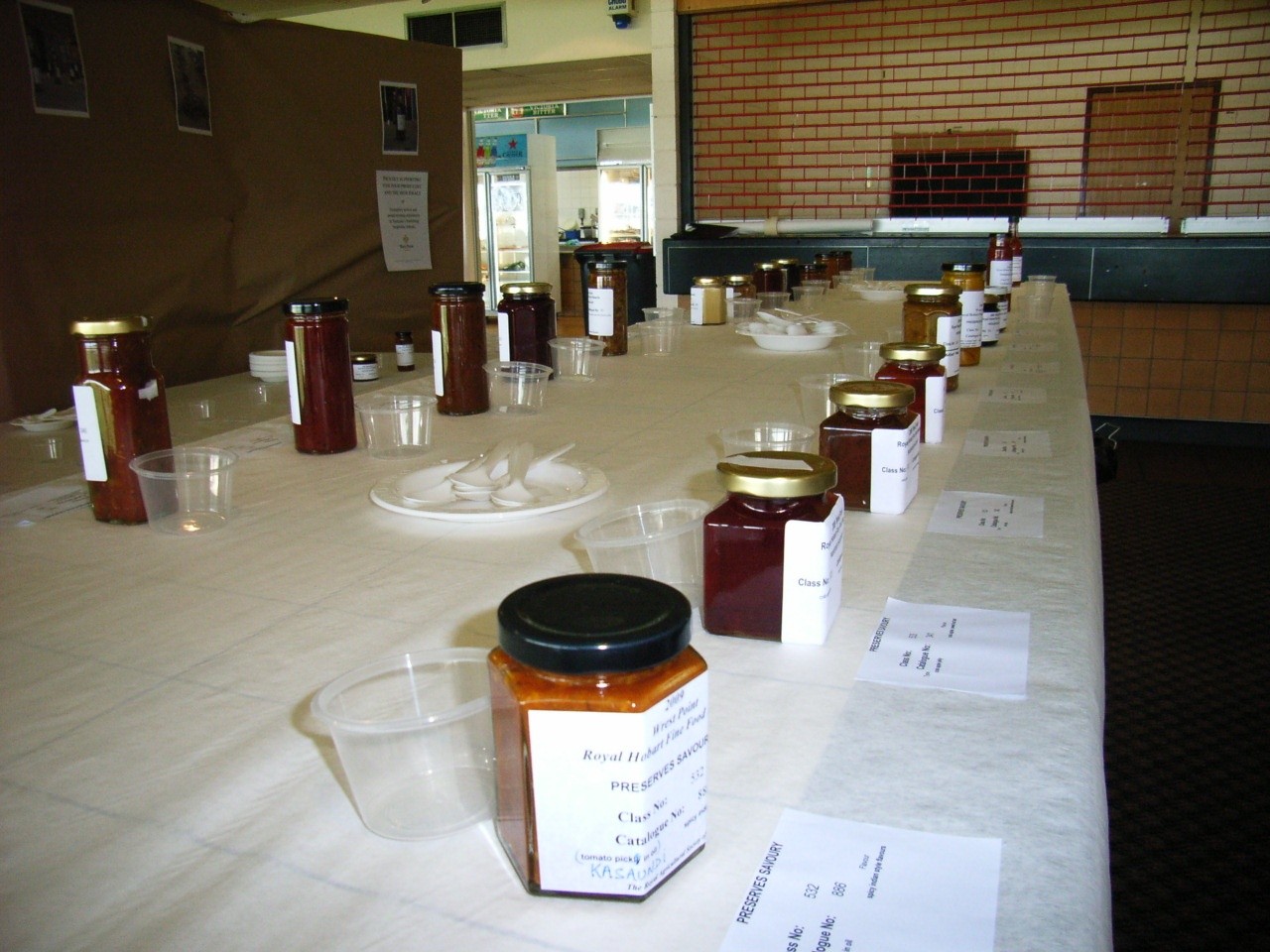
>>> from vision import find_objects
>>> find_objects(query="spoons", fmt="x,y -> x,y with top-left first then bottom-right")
404,437 -> 576,505
749,308 -> 838,335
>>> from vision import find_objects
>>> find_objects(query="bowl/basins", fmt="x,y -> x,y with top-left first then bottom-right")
1016,295 -> 1054,318
190,399 -> 221,420
841,341 -> 885,381
886,328 -> 902,342
723,292 -> 791,325
354,394 -> 438,460
29,438 -> 66,461
548,338 -> 607,384
636,307 -> 684,357
310,646 -> 496,841
573,497 -> 713,608
255,383 -> 280,403
797,373 -> 867,427
717,422 -> 814,456
791,267 -> 874,306
1024,275 -> 1057,296
482,361 -> 553,416
128,447 -> 238,534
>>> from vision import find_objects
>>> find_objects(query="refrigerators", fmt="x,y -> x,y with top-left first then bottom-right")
475,135 -> 562,319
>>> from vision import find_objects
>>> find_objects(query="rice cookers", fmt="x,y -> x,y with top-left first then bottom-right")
580,226 -> 599,242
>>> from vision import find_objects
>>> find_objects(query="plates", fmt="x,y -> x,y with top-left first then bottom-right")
857,290 -> 907,300
10,419 -> 74,431
370,456 -> 609,522
248,350 -> 288,383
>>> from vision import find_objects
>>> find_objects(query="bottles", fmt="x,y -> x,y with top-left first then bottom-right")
987,232 -> 1013,289
1007,215 -> 1023,287
69,314 -> 178,524
395,331 -> 415,372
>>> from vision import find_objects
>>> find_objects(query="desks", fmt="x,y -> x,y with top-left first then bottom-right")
0,271 -> 1120,952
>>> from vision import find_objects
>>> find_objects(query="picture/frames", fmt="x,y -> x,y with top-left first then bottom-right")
163,33 -> 216,138
14,0 -> 93,121
376,78 -> 423,158
